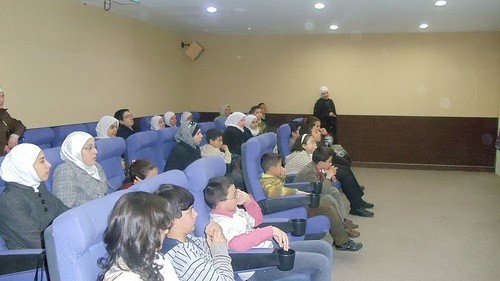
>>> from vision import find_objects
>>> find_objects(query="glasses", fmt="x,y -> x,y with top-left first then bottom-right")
83,145 -> 98,150
219,193 -> 238,203
123,114 -> 134,120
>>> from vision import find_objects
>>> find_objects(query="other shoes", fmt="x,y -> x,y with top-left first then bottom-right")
335,239 -> 363,251
360,200 -> 374,208
343,228 -> 359,238
360,186 -> 365,191
349,208 -> 374,217
344,218 -> 359,229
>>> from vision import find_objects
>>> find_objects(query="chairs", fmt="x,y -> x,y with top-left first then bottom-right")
1,109 -> 342,281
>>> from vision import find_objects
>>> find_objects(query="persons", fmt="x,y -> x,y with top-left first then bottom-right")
0,89 -> 26,157
199,128 -> 245,187
213,102 -> 267,154
313,87 -> 337,145
284,116 -> 375,217
96,190 -> 179,281
113,109 -> 141,140
96,116 -> 119,139
259,152 -> 362,251
203,176 -> 333,281
292,146 -> 360,238
0,143 -> 71,250
120,160 -> 159,190
153,183 -> 235,281
164,122 -> 203,172
51,131 -> 117,209
150,111 -> 193,131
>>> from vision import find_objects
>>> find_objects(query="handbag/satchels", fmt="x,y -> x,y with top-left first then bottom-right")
331,145 -> 348,158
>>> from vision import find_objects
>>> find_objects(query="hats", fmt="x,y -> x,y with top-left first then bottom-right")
189,122 -> 200,137
